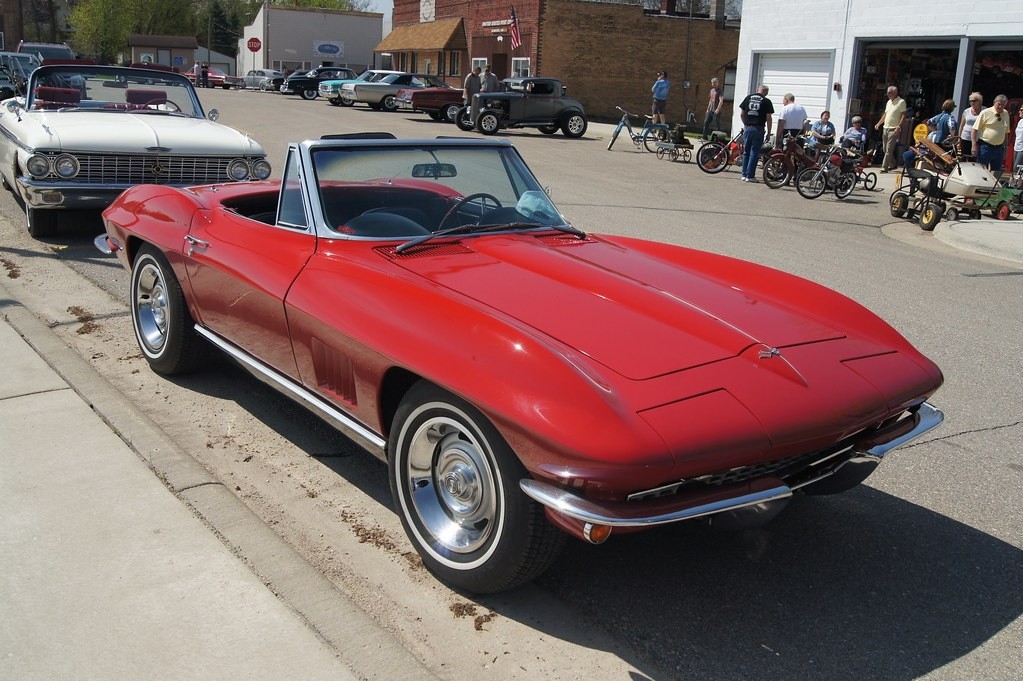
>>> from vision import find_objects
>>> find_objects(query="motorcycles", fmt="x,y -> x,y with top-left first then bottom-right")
696,119 -> 877,200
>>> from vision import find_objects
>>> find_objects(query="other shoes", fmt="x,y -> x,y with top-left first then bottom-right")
699,138 -> 707,142
880,168 -> 888,173
741,176 -> 746,181
650,129 -> 656,133
888,165 -> 897,171
745,177 -> 759,183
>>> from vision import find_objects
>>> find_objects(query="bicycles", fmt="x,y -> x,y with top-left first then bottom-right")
606,105 -> 670,154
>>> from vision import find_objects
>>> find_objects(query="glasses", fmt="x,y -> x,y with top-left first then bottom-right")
954,105 -> 957,107
996,113 -> 1001,121
969,99 -> 979,102
854,122 -> 860,123
485,69 -> 490,72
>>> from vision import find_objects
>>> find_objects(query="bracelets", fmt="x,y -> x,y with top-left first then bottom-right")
897,126 -> 900,128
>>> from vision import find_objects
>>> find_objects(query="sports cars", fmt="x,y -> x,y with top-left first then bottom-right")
0,60 -> 272,239
94,132 -> 945,600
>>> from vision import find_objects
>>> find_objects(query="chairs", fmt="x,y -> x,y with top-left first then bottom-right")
36,87 -> 81,110
125,90 -> 167,110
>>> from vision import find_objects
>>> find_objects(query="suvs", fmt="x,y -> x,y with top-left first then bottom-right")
18,39 -> 87,100
128,62 -> 173,86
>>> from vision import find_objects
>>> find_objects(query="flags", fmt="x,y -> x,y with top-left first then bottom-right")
511,6 -> 521,50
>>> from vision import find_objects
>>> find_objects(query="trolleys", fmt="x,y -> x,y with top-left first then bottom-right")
655,141 -> 694,163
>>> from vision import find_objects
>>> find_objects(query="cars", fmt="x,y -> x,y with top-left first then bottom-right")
454,78 -> 588,139
318,69 -> 425,107
286,65 -> 359,100
238,70 -> 284,92
339,73 -> 450,112
279,69 -> 338,95
179,66 -> 230,90
392,88 -> 464,124
0,51 -> 45,102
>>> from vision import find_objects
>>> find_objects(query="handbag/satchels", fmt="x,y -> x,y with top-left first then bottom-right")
818,134 -> 834,144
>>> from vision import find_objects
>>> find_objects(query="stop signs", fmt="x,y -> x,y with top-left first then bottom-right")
247,38 -> 261,52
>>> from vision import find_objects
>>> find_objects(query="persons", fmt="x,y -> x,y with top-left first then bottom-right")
652,71 -> 670,133
841,116 -> 867,150
776,93 -> 808,186
1013,105 -> 1023,174
811,111 -> 835,145
875,85 -> 907,173
739,85 -> 774,182
957,92 -> 987,162
699,78 -> 723,142
479,64 -> 499,92
201,62 -> 209,88
903,119 -> 937,171
194,61 -> 200,87
927,100 -> 959,143
971,94 -> 1010,178
462,67 -> 481,106
